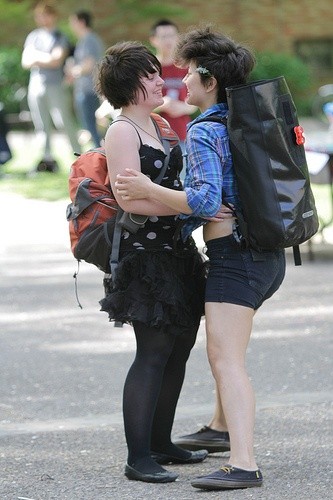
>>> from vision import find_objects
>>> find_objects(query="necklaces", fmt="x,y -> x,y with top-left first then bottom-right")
119,114 -> 161,144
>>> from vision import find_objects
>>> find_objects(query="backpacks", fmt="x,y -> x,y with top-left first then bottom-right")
187,75 -> 319,253
66,111 -> 182,274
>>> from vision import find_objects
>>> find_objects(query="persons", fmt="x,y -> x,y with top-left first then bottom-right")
96,40 -> 235,482
149,19 -> 199,187
22,4 -> 84,172
64,11 -> 107,150
114,25 -> 286,488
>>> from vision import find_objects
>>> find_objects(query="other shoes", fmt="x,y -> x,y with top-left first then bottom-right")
37,160 -> 56,171
124,463 -> 178,483
151,449 -> 208,465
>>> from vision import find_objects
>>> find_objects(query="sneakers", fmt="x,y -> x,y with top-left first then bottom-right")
172,425 -> 231,453
191,463 -> 263,489
